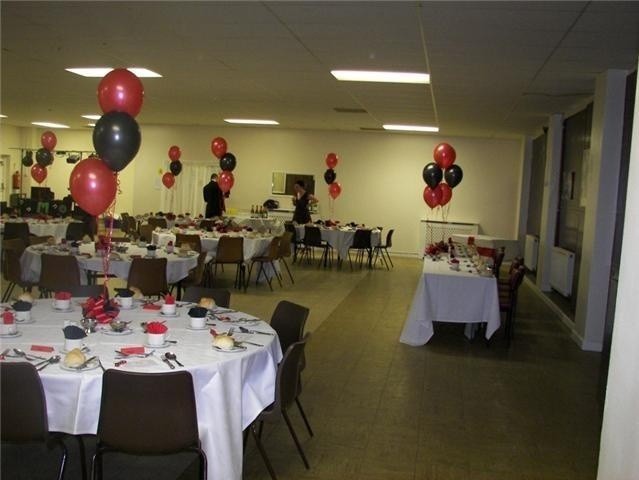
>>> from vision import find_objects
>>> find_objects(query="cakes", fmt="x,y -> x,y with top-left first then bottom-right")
108,253 -> 120,259
178,249 -> 188,257
82,235 -> 91,243
181,244 -> 191,251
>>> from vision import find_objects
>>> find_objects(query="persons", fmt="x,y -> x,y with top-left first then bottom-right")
291,179 -> 320,225
203,173 -> 226,219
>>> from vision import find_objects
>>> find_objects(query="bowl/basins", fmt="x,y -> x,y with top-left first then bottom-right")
476,266 -> 493,277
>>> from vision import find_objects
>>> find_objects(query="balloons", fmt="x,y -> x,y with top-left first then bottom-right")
98,68 -> 145,119
69,158 -> 118,222
211,137 -> 228,159
324,168 -> 336,185
36,148 -> 52,168
325,152 -> 338,169
41,130 -> 57,152
329,182 -> 342,200
218,171 -> 235,193
162,172 -> 175,189
31,163 -> 47,184
92,110 -> 142,176
219,152 -> 237,172
423,162 -> 443,191
168,145 -> 181,161
444,164 -> 464,189
170,159 -> 183,176
433,143 -> 456,169
439,183 -> 452,208
423,184 -> 443,210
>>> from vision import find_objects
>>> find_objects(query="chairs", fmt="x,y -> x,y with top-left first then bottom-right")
182,285 -> 230,309
367,229 -> 395,271
29,234 -> 53,244
92,369 -> 208,479
65,220 -> 95,242
246,237 -> 284,292
347,229 -> 373,270
281,232 -> 297,286
1,238 -> 29,303
270,299 -> 315,438
300,226 -> 329,268
129,257 -> 172,300
1,361 -> 88,480
3,222 -> 32,241
478,243 -> 526,347
38,252 -> 82,297
245,331 -> 315,480
71,283 -> 110,300
209,235 -> 248,291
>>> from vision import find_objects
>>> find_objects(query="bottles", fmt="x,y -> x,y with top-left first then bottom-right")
250,204 -> 254,218
262,203 -> 267,218
258,205 -> 262,218
254,204 -> 258,218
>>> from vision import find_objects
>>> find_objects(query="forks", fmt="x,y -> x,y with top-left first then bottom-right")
227,327 -> 234,336
161,355 -> 175,369
1,348 -> 11,356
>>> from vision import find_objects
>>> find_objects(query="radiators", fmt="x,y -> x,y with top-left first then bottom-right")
549,246 -> 575,299
525,234 -> 538,273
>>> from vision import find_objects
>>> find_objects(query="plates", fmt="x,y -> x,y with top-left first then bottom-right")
143,341 -> 170,348
60,354 -> 100,371
195,304 -> 218,311
211,343 -> 246,353
102,327 -> 132,336
0,330 -> 23,338
143,254 -> 158,259
117,305 -> 140,313
9,300 -> 37,306
58,342 -> 87,354
14,318 -> 35,325
49,306 -> 74,312
160,310 -> 179,317
185,324 -> 209,330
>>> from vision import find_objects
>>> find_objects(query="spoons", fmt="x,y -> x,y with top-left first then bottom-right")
233,340 -> 264,347
4,348 -> 46,361
208,308 -> 232,312
239,326 -> 274,335
239,317 -> 261,323
165,351 -> 184,367
176,302 -> 192,307
33,355 -> 61,371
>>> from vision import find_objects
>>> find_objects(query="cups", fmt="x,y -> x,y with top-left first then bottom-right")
63,338 -> 82,351
97,249 -> 105,256
162,304 -> 176,314
166,244 -> 173,253
14,310 -> 31,322
190,318 -> 205,328
451,262 -> 458,270
246,233 -> 257,239
352,227 -> 356,231
147,249 -> 155,257
71,246 -> 78,253
117,297 -> 132,309
213,230 -> 218,238
332,226 -> 337,231
188,226 -> 195,231
0,323 -> 17,335
108,320 -> 126,332
173,227 -> 180,235
147,332 -> 165,345
80,318 -> 98,334
51,298 -> 70,309
242,228 -> 248,234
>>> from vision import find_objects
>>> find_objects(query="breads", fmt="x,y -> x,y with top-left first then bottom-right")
211,334 -> 233,350
198,296 -> 215,310
47,236 -> 56,246
63,348 -> 85,368
17,291 -> 33,304
